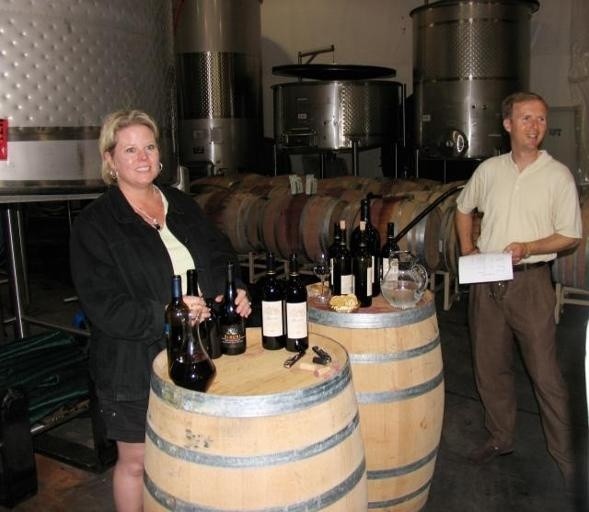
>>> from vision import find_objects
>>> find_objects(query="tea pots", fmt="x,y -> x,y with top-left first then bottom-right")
380,251 -> 428,310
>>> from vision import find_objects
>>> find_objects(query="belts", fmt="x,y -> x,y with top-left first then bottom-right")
513,262 -> 545,272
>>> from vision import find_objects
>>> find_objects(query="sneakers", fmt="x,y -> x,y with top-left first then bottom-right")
468,436 -> 514,466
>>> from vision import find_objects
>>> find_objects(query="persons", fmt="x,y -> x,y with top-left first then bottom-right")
451,90 -> 583,501
71,108 -> 252,512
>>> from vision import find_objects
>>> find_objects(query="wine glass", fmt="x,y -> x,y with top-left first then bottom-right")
314,252 -> 330,304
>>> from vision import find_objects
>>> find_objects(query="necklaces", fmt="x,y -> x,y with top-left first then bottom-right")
125,200 -> 164,232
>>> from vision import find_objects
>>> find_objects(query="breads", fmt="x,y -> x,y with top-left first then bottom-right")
307,284 -> 362,312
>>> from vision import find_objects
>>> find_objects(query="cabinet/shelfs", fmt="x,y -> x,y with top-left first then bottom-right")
0,184 -> 117,508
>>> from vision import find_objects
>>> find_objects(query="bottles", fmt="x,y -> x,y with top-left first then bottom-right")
283,252 -> 309,353
165,273 -> 185,375
203,303 -> 222,359
168,315 -> 217,393
330,198 -> 399,308
220,261 -> 247,356
259,251 -> 284,350
185,267 -> 210,360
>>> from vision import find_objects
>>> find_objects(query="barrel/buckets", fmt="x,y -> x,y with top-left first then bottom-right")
185,174 -> 482,275
187,190 -> 269,255
551,186 -> 588,287
437,208 -> 480,273
246,193 -> 345,264
338,193 -> 445,269
